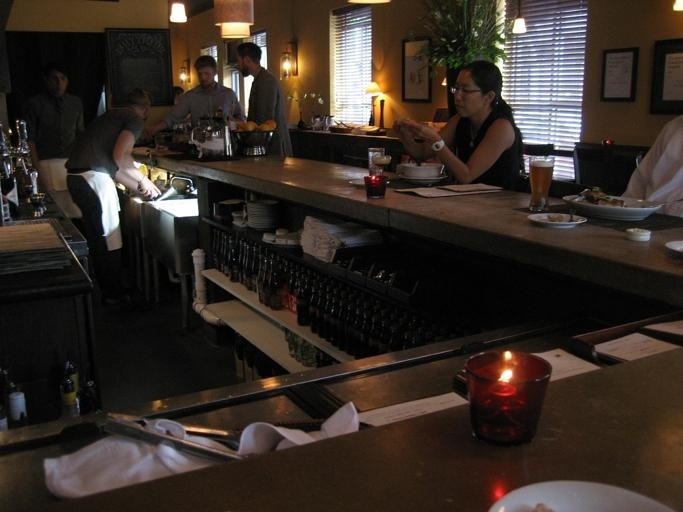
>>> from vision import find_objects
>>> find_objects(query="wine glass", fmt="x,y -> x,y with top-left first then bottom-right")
372,155 -> 392,174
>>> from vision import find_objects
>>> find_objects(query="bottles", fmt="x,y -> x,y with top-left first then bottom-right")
211,229 -> 461,360
195,119 -> 201,129
172,122 -> 177,144
178,122 -> 184,144
62,377 -> 78,420
186,121 -> 192,145
225,126 -> 233,158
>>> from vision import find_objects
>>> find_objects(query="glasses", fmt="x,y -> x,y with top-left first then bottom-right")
450,86 -> 481,94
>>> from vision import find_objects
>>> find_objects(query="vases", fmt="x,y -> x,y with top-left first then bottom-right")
298,111 -> 306,129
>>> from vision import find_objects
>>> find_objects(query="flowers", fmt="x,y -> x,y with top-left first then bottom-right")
286,91 -> 324,111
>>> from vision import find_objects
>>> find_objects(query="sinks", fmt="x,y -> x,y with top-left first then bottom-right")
127,188 -> 174,204
143,198 -> 199,218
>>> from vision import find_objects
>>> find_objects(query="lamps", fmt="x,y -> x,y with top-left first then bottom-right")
212,0 -> 256,39
366,82 -> 385,97
278,47 -> 293,82
176,65 -> 189,86
511,0 -> 528,36
168,2 -> 188,24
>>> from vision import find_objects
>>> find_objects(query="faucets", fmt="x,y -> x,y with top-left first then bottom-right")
169,176 -> 193,193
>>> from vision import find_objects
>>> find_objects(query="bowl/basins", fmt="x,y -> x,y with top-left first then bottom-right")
398,162 -> 445,178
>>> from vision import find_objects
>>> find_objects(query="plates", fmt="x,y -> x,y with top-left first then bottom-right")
487,479 -> 673,512
527,211 -> 589,230
397,173 -> 450,186
245,199 -> 280,233
665,240 -> 683,254
562,193 -> 667,222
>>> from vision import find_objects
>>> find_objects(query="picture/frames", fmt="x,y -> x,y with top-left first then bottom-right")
401,35 -> 434,104
600,46 -> 639,102
106,27 -> 174,111
649,38 -> 683,116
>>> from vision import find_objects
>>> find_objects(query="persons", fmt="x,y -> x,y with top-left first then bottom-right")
145,55 -> 243,156
622,113 -> 683,218
236,42 -> 292,157
393,59 -> 524,191
174,86 -> 184,104
23,63 -> 84,220
64,88 -> 162,305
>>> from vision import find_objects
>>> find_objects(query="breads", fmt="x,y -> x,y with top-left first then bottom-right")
598,196 -> 624,207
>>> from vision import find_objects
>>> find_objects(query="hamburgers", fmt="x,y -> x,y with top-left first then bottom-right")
585,187 -> 604,205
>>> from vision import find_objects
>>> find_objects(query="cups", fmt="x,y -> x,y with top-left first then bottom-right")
367,147 -> 385,174
363,174 -> 388,199
527,154 -> 555,212
464,349 -> 553,449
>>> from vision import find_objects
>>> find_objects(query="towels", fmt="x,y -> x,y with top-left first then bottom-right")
41,397 -> 359,499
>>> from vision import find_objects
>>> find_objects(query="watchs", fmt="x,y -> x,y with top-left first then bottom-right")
431,139 -> 444,152
